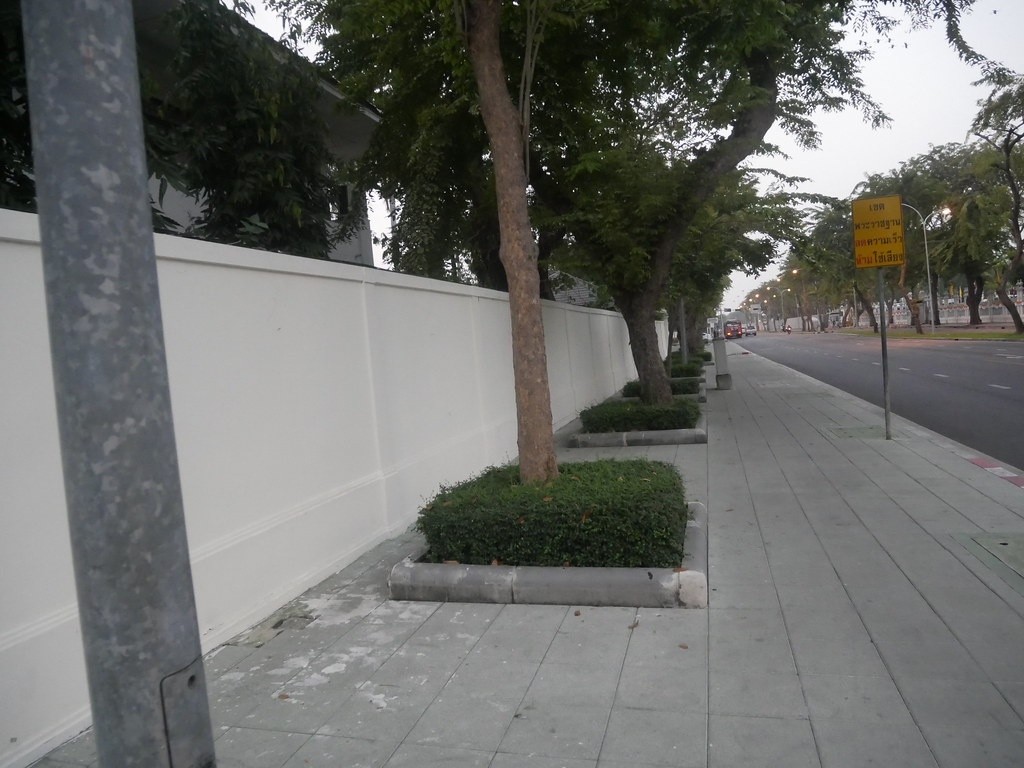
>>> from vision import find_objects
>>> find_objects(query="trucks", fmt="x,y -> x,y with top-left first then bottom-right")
724,319 -> 742,339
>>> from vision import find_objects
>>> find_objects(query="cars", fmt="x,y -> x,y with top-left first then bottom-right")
702,333 -> 714,343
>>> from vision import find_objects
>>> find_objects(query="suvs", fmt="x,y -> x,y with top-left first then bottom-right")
746,326 -> 756,337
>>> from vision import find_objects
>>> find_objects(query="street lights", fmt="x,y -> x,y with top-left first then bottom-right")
790,269 -> 810,330
766,286 -> 791,328
901,202 -> 951,334
